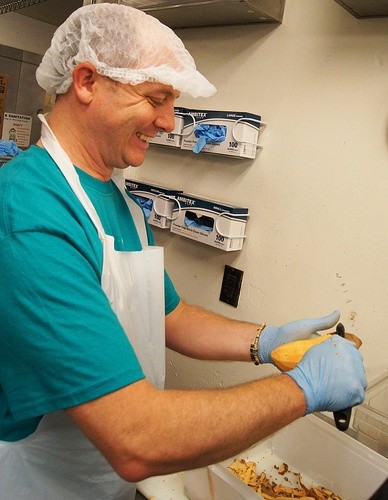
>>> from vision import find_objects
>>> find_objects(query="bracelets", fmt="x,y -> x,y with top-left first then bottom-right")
249,325 -> 266,366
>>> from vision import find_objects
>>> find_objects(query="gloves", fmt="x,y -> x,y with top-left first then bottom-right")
258,310 -> 341,364
282,334 -> 368,417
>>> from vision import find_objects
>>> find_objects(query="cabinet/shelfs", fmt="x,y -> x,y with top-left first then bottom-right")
82,0 -> 286,28
0,44 -> 45,165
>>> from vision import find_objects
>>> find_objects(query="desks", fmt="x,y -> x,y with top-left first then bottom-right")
133,366 -> 388,500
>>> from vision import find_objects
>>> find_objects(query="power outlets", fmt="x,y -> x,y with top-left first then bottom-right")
219,264 -> 243,308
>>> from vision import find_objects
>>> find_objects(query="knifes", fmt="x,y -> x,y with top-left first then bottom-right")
332,323 -> 354,430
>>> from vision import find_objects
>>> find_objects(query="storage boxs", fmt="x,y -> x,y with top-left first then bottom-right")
183,413 -> 388,499
149,106 -> 192,151
180,109 -> 261,160
353,369 -> 388,459
170,193 -> 248,252
124,177 -> 184,228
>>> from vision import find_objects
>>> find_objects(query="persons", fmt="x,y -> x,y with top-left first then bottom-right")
0,3 -> 367,500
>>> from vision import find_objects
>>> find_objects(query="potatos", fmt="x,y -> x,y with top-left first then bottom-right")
270,331 -> 364,372
